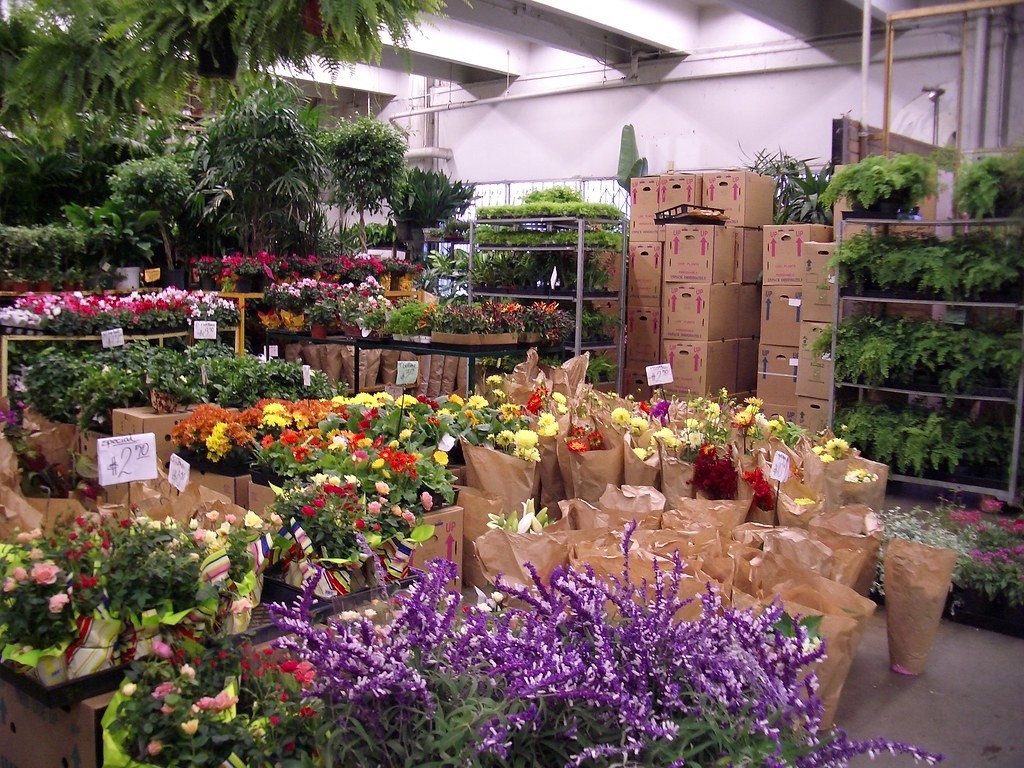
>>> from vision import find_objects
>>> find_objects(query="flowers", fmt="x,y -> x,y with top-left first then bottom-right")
1,253 -> 1024,767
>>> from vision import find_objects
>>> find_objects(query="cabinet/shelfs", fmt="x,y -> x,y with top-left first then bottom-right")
198,287 -> 425,362
827,216 -> 1024,508
468,219 -> 627,394
1,326 -> 239,403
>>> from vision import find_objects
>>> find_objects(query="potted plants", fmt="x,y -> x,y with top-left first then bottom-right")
87,199 -> 156,293
829,307 -> 915,390
883,406 -> 948,481
951,230 -> 1023,301
935,310 -> 1022,399
30,263 -> 60,292
942,414 -> 1016,488
892,236 -> 956,296
815,147 -> 937,217
385,170 -> 478,240
3,262 -> 40,294
53,266 -> 84,290
954,147 -> 1023,218
836,402 -> 898,474
835,229 -> 901,298
908,317 -> 974,394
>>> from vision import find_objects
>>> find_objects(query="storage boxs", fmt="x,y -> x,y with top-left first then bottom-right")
412,505 -> 468,591
589,348 -> 623,393
109,403 -> 230,461
702,174 -> 775,227
631,175 -> 663,221
583,251 -> 624,296
179,466 -> 253,519
893,169 -> 952,241
831,162 -> 889,232
78,426 -> 106,461
628,223 -> 947,437
660,176 -> 705,215
0,679 -> 117,768
105,481 -> 130,509
247,479 -> 283,524
128,468 -> 178,521
586,302 -> 619,344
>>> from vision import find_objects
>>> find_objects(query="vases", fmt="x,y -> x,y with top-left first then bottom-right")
180,446 -> 251,477
245,466 -> 285,489
412,483 -> 459,509
